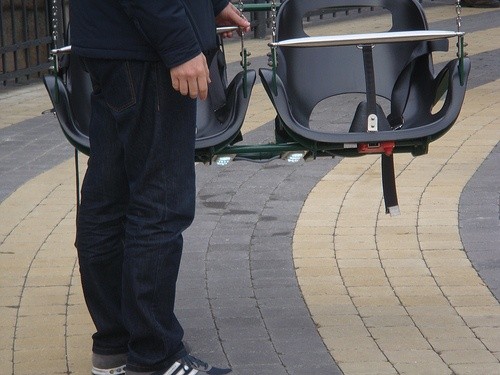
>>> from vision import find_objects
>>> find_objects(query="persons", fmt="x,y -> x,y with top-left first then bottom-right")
67,0 -> 251,375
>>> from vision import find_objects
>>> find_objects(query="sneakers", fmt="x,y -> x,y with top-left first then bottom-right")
128,344 -> 234,375
90,351 -> 128,375
